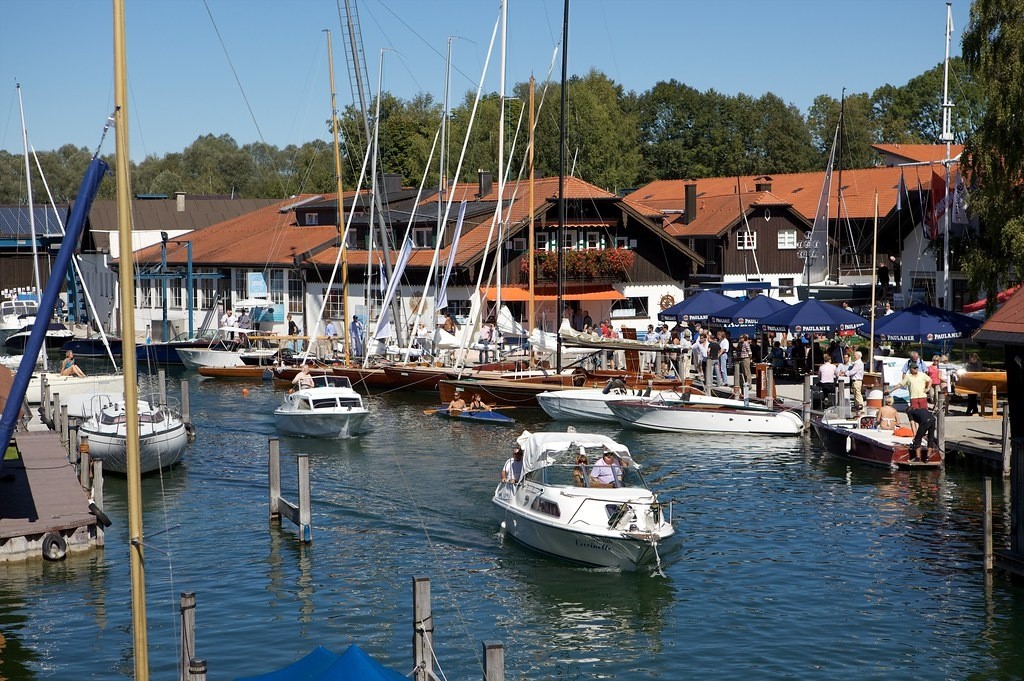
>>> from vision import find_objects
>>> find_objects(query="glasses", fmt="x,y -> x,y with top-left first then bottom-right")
604,453 -> 613,456
578,460 -> 586,464
454,395 -> 459,397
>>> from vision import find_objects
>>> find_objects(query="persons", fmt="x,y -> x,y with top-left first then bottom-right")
219,308 -> 250,340
843,256 -> 902,321
287,314 -> 299,350
349,315 -> 363,358
448,391 -> 487,411
643,320 -> 737,387
408,311 -> 455,362
292,363 -> 315,390
500,446 -> 629,489
60,349 -> 89,379
477,318 -> 498,364
876,351 -> 982,463
734,333 -> 847,391
562,305 -> 620,370
324,318 -> 337,356
837,342 -> 890,410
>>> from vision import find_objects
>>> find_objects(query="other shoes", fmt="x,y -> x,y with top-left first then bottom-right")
962,411 -> 971,416
924,456 -> 930,463
908,458 -> 920,463
945,412 -> 954,416
972,409 -> 978,414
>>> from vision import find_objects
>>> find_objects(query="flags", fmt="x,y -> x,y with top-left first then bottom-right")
895,169 -> 983,243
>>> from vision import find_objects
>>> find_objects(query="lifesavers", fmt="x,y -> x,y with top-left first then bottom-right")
89,503 -> 112,527
43,534 -> 66,559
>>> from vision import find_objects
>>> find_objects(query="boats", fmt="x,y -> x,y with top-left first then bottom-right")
274,374 -> 371,438
494,429 -> 681,578
426,402 -> 513,428
952,370 -> 1007,398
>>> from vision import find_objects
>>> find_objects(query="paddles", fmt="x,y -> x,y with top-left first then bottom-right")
423,406 -> 517,414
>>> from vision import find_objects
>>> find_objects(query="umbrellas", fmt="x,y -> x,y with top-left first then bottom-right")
709,296 -> 792,360
754,298 -> 867,371
657,291 -> 740,327
855,302 -> 983,357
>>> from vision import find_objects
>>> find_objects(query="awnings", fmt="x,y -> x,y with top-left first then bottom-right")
480,284 -> 628,302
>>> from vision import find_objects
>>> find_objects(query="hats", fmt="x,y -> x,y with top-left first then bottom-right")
910,363 -> 918,368
603,447 -> 613,454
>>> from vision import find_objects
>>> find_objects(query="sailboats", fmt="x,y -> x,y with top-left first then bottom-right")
812,191 -> 949,473
0,0 -> 807,485
